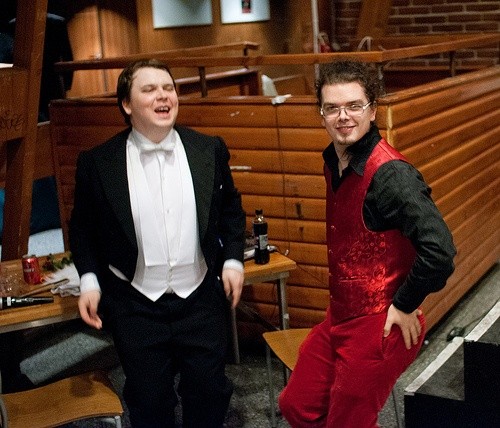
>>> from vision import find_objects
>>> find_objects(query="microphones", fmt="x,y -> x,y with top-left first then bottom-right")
0,296 -> 54,309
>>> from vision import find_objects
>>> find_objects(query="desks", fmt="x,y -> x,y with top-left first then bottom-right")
0,250 -> 297,388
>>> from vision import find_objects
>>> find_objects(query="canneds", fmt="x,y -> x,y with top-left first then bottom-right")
22,255 -> 41,285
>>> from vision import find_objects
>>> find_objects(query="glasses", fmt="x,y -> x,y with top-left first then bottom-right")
320,102 -> 371,118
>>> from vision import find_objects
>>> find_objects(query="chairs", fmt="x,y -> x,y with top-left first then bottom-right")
262,328 -> 400,428
0,370 -> 124,428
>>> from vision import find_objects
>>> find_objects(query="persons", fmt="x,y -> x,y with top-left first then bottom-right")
278,53 -> 457,428
69,60 -> 247,428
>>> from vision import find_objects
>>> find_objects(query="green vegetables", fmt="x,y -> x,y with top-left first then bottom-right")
41,253 -> 73,272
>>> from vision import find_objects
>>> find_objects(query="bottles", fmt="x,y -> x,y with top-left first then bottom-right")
252,207 -> 270,264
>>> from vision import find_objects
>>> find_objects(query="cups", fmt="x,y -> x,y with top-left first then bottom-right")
0,271 -> 26,297
